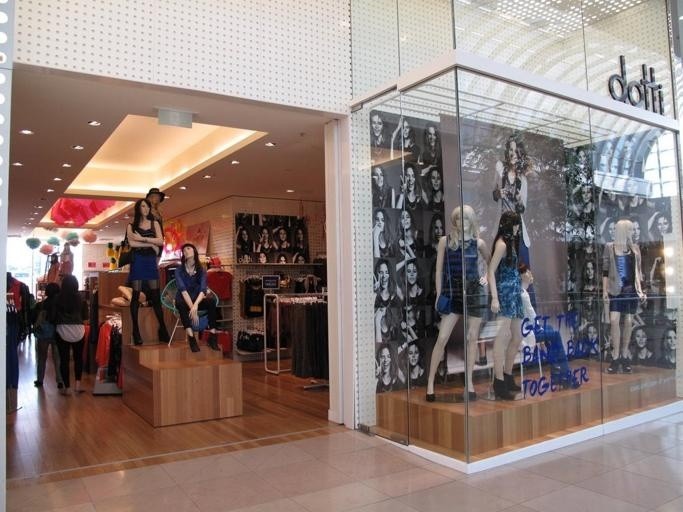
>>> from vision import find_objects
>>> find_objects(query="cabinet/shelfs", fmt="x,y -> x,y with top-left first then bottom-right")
97,271 -> 243,428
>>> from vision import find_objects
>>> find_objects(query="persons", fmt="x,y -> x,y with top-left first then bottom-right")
567,139 -> 676,367
31,283 -> 63,388
6,272 -> 31,414
371,110 -> 444,394
49,275 -> 89,395
111,286 -> 153,307
146,188 -> 165,265
425,205 -> 526,402
235,226 -> 308,263
491,133 -> 536,307
174,244 -> 221,352
600,219 -> 645,376
127,198 -> 172,345
47,254 -> 60,283
60,243 -> 73,277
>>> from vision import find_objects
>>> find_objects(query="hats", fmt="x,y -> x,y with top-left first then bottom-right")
146,188 -> 165,202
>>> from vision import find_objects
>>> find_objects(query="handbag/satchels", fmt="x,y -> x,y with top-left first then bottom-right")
437,295 -> 451,313
33,311 -> 54,339
118,241 -> 131,267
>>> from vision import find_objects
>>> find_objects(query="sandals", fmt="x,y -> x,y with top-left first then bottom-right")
608,356 -> 620,373
620,354 -> 632,372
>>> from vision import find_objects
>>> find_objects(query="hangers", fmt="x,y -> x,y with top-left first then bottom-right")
273,293 -> 328,304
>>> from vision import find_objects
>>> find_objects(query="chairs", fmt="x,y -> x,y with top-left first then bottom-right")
503,319 -> 544,392
161,277 -> 220,347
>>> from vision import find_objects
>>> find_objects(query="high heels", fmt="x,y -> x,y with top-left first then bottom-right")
494,376 -> 514,399
504,372 -> 521,390
133,328 -> 143,343
188,335 -> 200,352
158,325 -> 172,343
207,332 -> 221,350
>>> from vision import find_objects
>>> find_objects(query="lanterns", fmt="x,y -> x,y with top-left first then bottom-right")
26,232 -> 97,255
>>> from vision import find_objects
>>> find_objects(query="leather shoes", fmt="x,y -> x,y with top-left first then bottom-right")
469,390 -> 477,400
34,381 -> 85,395
426,381 -> 435,401
